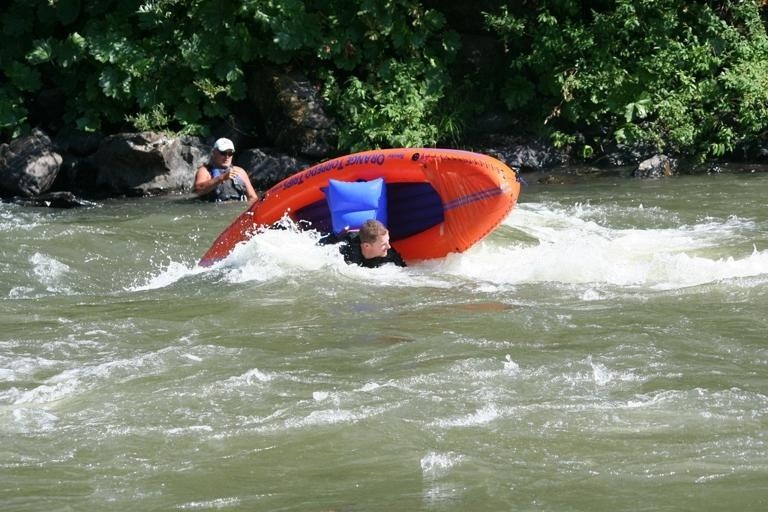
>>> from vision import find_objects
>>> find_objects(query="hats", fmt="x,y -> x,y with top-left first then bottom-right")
212,137 -> 235,152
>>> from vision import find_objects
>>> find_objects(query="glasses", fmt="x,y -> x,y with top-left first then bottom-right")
217,149 -> 234,156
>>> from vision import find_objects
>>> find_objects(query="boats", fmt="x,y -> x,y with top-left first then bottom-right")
196,145 -> 517,274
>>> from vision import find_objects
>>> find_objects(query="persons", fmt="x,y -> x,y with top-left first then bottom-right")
331,217 -> 407,270
192,136 -> 259,205
